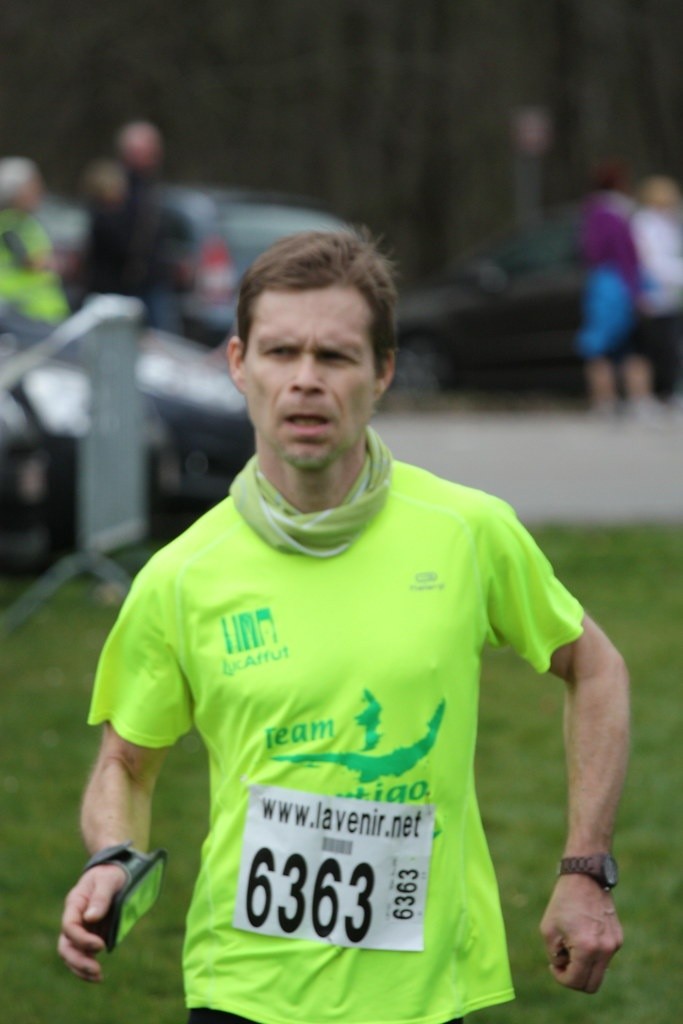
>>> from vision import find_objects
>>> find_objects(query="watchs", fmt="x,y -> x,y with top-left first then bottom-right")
559,852 -> 619,892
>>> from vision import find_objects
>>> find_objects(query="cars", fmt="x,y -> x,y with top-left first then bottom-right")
0,188 -> 357,578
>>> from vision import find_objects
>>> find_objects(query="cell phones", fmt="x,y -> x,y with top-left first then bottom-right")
105,846 -> 168,956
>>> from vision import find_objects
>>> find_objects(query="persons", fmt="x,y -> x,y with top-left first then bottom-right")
0,121 -> 200,339
573,160 -> 683,420
55,231 -> 630,1024
